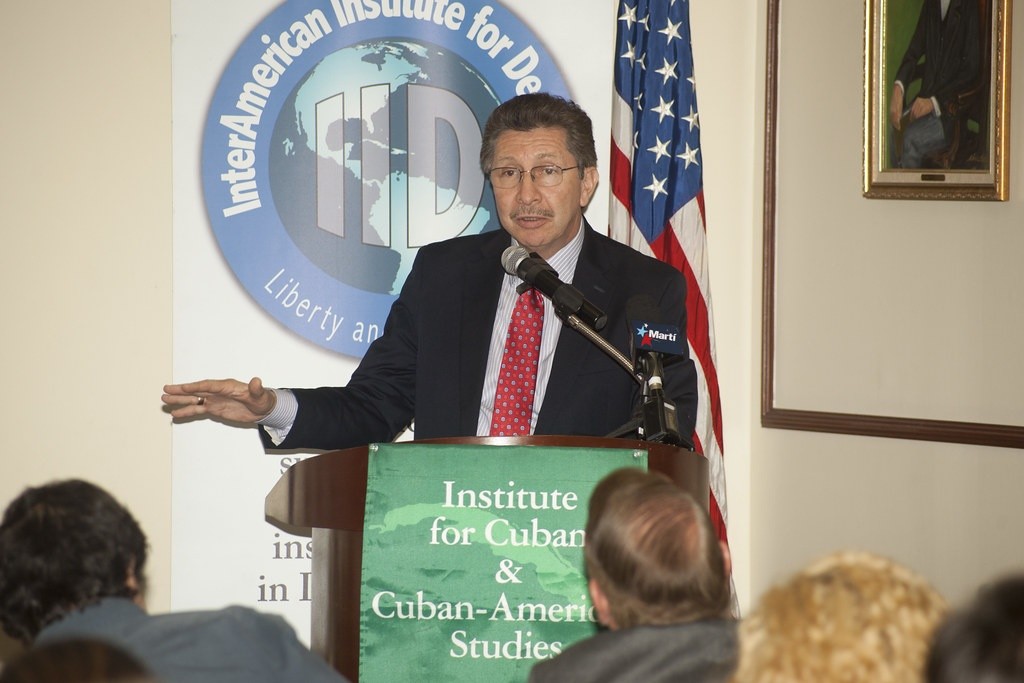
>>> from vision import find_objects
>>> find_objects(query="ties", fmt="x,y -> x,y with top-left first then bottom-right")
490,286 -> 545,439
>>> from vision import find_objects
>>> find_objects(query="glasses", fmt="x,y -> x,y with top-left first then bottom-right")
488,163 -> 595,189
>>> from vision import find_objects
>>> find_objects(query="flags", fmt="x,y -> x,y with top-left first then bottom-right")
610,1 -> 738,603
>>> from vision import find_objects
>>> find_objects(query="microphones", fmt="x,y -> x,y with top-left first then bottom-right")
501,245 -> 609,332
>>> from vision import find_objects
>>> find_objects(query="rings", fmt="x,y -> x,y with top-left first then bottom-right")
197,396 -> 205,405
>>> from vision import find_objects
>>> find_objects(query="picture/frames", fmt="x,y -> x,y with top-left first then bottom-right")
863,0 -> 1012,203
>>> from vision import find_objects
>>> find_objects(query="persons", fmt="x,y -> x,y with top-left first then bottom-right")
735,557 -> 947,683
4,482 -> 355,683
923,572 -> 1024,683
161,94 -> 697,447
528,465 -> 739,682
